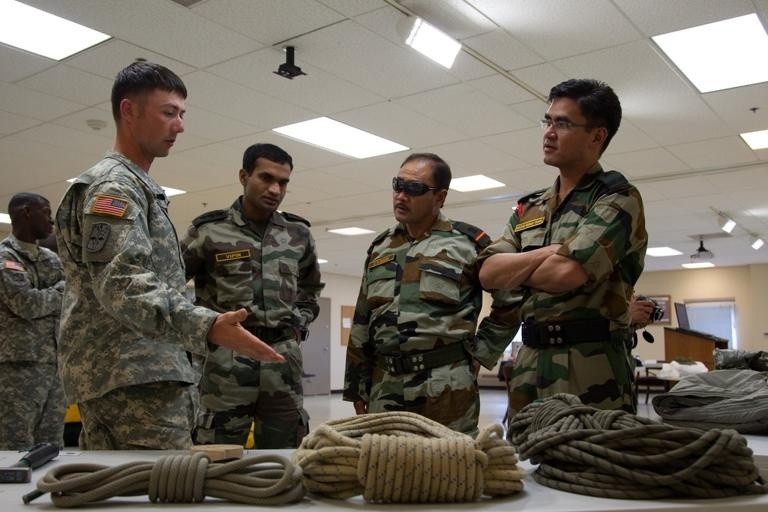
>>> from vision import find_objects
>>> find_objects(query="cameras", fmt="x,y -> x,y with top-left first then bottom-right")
636,294 -> 664,323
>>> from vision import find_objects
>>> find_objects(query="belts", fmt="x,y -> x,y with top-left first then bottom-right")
371,342 -> 468,377
246,326 -> 297,345
520,320 -> 611,350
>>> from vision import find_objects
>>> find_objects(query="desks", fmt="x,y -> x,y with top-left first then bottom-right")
0,432 -> 768,512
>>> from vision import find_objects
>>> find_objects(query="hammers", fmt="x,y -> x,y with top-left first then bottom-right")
0,442 -> 60,483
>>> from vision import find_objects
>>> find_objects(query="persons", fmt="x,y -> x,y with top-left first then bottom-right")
339,151 -> 526,444
52,56 -> 287,451
180,142 -> 327,448
0,191 -> 69,453
628,288 -> 655,352
474,76 -> 648,426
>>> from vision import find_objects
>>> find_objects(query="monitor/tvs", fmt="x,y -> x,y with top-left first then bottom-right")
675,302 -> 690,329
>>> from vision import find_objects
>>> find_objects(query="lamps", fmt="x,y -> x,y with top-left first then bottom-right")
689,239 -> 714,260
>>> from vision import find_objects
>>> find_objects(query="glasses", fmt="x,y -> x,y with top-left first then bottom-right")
391,177 -> 438,197
540,119 -> 591,131
